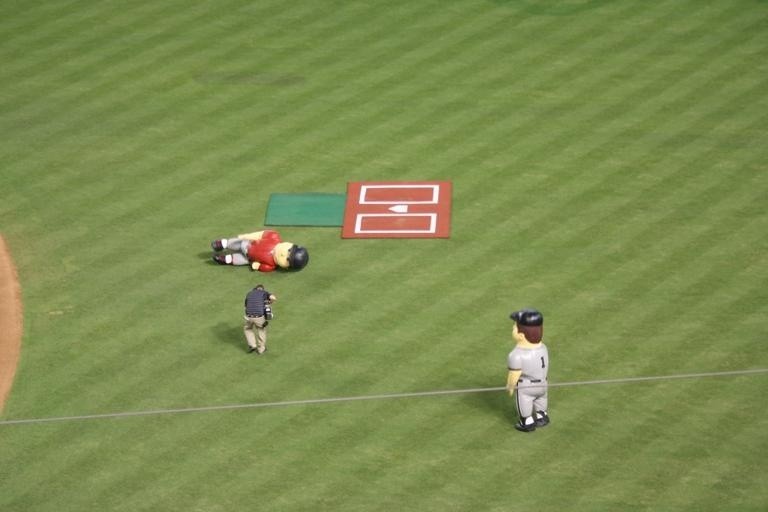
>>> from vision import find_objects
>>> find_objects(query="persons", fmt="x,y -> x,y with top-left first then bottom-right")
242,285 -> 277,354
504,308 -> 550,433
210,230 -> 308,273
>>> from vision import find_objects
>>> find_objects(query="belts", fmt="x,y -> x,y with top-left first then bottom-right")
248,315 -> 262,317
